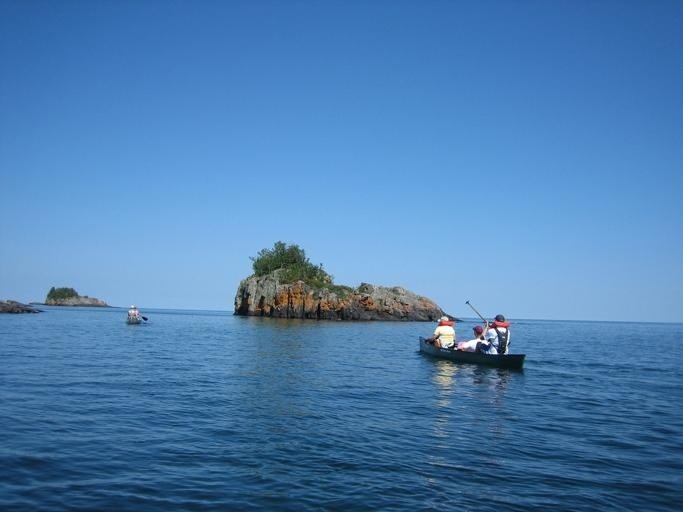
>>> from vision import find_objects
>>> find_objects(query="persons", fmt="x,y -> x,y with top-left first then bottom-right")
425,316 -> 455,348
454,326 -> 492,353
482,314 -> 510,354
128,305 -> 139,318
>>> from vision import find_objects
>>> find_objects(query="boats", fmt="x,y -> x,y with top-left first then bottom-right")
127,317 -> 143,324
417,335 -> 527,370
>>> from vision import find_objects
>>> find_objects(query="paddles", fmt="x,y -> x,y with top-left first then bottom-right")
139,314 -> 148,321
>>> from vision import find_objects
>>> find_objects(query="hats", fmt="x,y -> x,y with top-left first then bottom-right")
473,325 -> 483,333
437,316 -> 448,322
495,314 -> 504,322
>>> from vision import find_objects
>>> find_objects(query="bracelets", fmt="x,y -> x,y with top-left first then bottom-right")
484,326 -> 488,331
